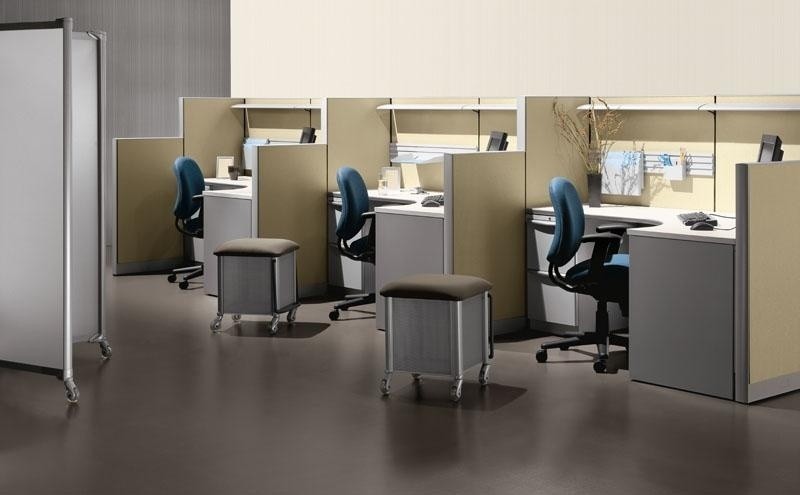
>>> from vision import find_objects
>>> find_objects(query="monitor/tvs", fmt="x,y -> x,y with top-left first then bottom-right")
486,131 -> 509,151
757,135 -> 784,161
300,127 -> 317,143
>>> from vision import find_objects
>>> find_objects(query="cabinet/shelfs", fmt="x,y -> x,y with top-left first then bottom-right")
209,238 -> 304,333
378,272 -> 497,401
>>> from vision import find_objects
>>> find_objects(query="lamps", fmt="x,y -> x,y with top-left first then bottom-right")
575,102 -> 800,115
376,103 -> 517,114
230,103 -> 323,112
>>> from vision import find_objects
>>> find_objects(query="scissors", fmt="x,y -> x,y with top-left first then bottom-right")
657,154 -> 671,166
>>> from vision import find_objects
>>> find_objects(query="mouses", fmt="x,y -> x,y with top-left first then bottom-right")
690,222 -> 713,230
422,200 -> 440,207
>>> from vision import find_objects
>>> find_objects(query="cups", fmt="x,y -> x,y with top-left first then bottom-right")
227,165 -> 239,181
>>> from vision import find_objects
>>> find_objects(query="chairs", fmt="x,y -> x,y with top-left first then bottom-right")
167,156 -> 217,289
537,177 -> 635,374
328,165 -> 388,326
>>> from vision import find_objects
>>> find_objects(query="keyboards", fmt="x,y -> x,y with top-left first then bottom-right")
421,195 -> 444,205
680,212 -> 717,226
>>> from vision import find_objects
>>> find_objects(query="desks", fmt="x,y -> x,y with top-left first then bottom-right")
329,186 -> 443,328
193,172 -> 252,295
525,197 -> 735,405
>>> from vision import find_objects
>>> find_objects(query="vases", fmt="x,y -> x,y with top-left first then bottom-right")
587,172 -> 603,207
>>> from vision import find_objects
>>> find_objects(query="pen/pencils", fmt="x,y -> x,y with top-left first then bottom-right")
676,161 -> 678,165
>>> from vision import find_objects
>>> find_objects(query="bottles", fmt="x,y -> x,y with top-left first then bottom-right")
376,180 -> 389,196
